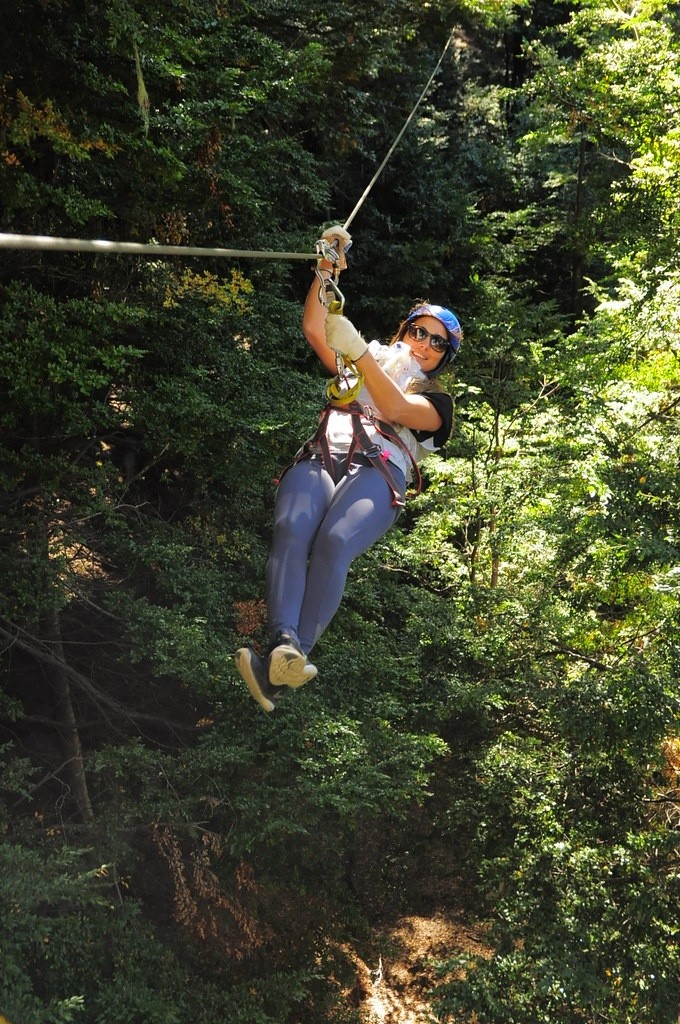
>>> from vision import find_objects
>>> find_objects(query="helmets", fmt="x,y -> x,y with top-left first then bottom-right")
398,304 -> 463,376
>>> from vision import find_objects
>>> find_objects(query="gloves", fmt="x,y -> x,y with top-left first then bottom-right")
317,224 -> 352,273
325,312 -> 370,363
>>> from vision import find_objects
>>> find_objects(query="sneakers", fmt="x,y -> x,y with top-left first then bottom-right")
269,632 -> 319,689
234,648 -> 287,714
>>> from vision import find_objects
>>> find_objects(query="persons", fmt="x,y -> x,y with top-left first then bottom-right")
235,225 -> 464,713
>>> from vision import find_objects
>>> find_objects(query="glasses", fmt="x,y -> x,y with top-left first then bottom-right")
407,322 -> 451,353
